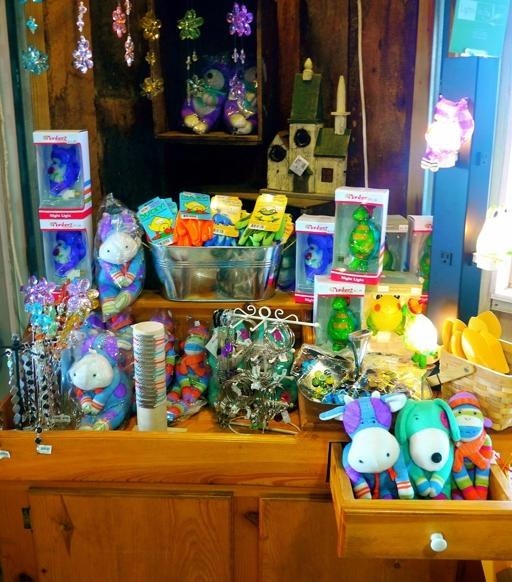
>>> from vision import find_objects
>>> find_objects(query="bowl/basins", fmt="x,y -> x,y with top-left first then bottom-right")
141,233 -> 297,302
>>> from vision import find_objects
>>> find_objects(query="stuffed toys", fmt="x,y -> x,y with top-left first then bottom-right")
450,390 -> 494,501
97,207 -> 147,318
150,313 -> 176,389
319,389 -> 408,499
167,321 -> 210,427
396,397 -> 463,501
65,328 -> 136,431
181,63 -> 230,133
225,53 -> 261,135
